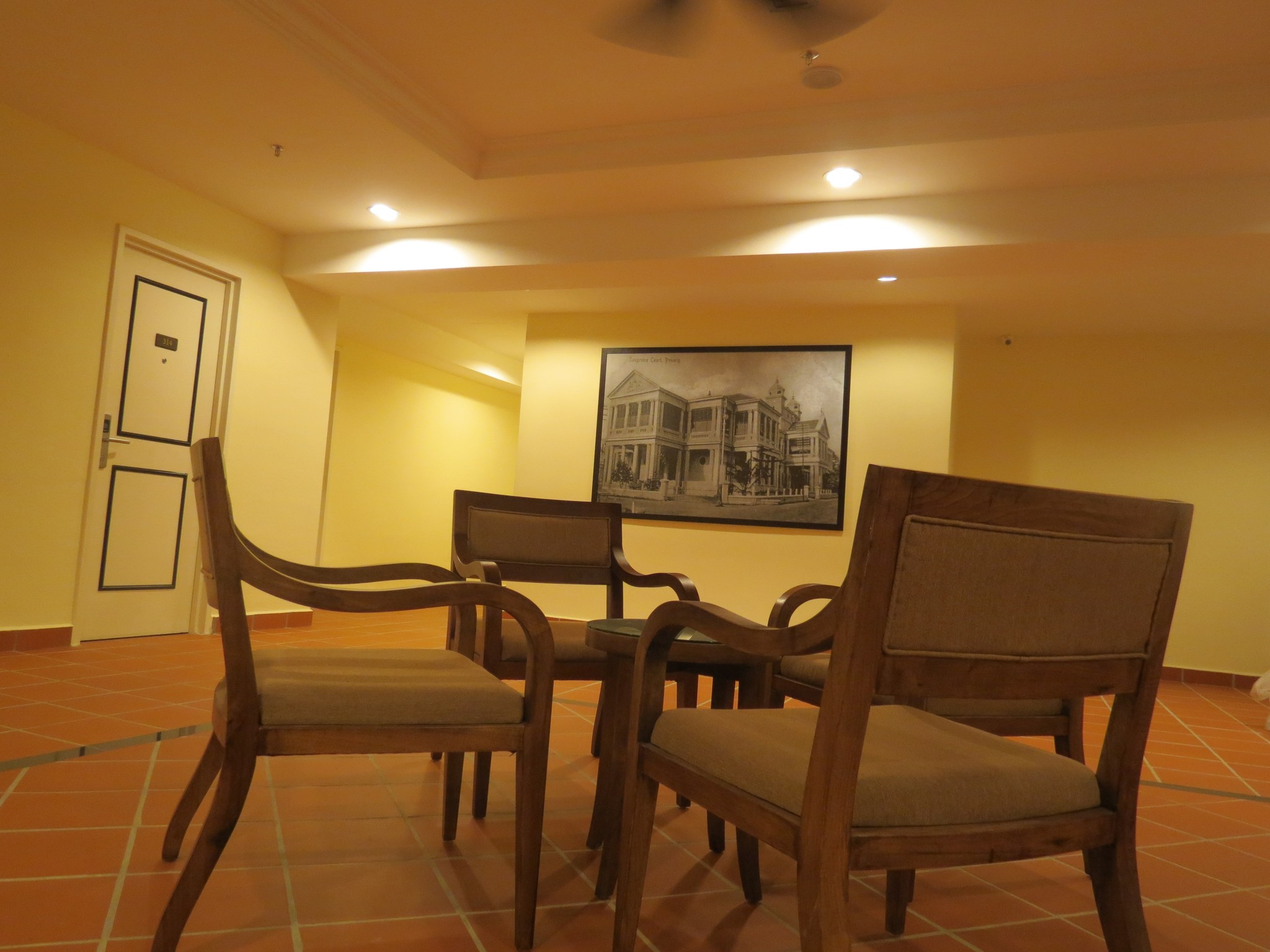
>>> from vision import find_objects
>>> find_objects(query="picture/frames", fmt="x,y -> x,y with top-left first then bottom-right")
592,344 -> 852,530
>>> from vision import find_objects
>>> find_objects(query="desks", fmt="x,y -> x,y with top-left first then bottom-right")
583,618 -> 762,908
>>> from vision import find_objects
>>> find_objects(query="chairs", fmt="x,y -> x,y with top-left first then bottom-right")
150,437 -> 556,952
430,489 -> 701,842
611,463 -> 1196,952
761,584 -> 1092,902
